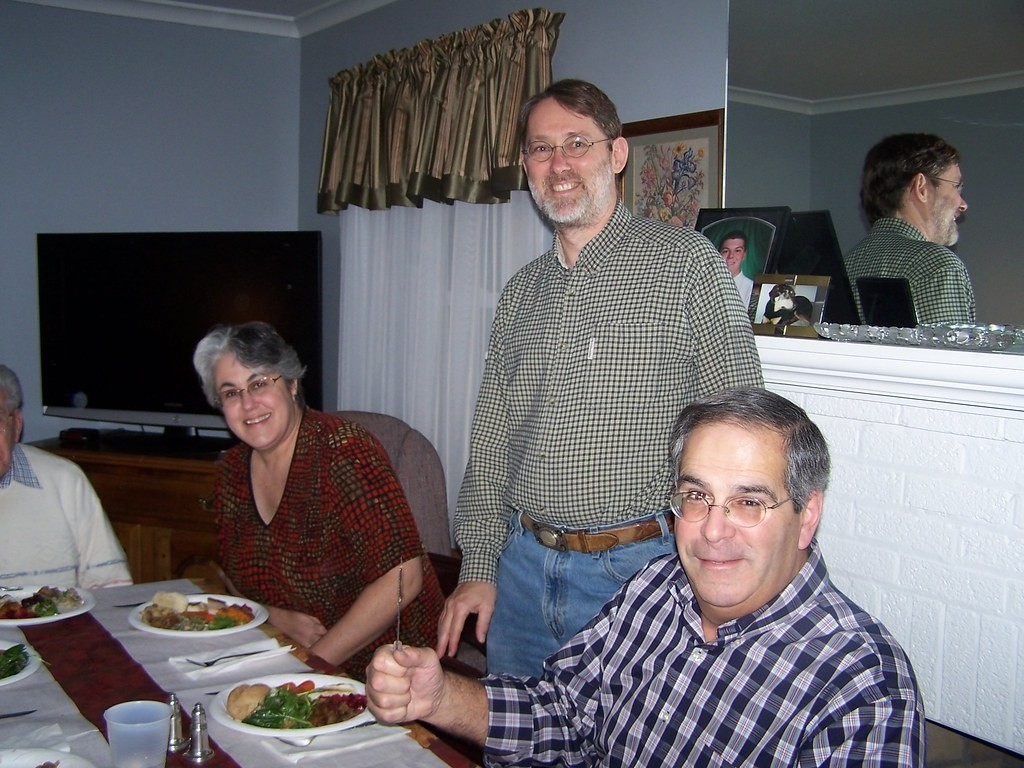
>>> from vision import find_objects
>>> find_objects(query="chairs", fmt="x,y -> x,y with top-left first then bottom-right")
332,409 -> 452,599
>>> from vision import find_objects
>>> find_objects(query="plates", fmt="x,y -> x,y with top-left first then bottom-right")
0,747 -> 95,767
0,637 -> 42,687
209,671 -> 376,736
128,593 -> 269,639
0,584 -> 98,626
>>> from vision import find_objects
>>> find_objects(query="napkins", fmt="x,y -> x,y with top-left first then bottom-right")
260,723 -> 412,764
0,722 -> 71,751
169,636 -> 297,682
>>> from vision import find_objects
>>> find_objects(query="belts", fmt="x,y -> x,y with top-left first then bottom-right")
520,513 -> 673,555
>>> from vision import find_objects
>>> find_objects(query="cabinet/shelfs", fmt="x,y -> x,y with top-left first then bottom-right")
23,427 -> 233,585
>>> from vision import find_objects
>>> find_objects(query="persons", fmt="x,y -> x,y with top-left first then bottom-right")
0,364 -> 134,589
718,231 -> 754,312
435,79 -> 767,678
845,133 -> 976,325
192,322 -> 446,679
365,383 -> 925,768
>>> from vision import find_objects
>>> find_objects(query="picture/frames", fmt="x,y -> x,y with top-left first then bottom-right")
747,273 -> 831,340
617,107 -> 722,233
693,205 -> 792,311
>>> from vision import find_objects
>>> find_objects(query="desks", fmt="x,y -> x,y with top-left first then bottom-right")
0,563 -> 483,767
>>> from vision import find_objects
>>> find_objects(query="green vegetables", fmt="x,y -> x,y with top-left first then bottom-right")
241,689 -> 315,729
0,644 -> 29,679
31,600 -> 58,616
207,613 -> 238,630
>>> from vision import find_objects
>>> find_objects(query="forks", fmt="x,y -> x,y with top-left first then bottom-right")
186,649 -> 270,668
273,721 -> 378,747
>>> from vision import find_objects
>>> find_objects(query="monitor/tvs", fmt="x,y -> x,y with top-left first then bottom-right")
37,230 -> 325,456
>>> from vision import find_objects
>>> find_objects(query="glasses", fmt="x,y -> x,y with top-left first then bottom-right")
521,136 -> 611,158
671,492 -> 790,527
217,376 -> 282,405
936,176 -> 964,194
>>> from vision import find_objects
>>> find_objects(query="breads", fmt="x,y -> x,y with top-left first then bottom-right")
226,682 -> 270,720
152,590 -> 189,613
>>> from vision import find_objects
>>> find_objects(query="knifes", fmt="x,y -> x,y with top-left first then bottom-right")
391,563 -> 403,652
205,692 -> 220,695
0,709 -> 37,719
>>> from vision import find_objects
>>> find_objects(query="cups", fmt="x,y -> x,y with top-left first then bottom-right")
103,699 -> 173,768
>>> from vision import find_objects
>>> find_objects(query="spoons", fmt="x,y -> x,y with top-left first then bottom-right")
0,584 -> 23,591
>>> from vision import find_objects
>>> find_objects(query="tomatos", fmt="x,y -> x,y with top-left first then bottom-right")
280,680 -> 315,694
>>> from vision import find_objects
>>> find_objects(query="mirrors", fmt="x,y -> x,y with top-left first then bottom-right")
722,0 -> 1024,356
